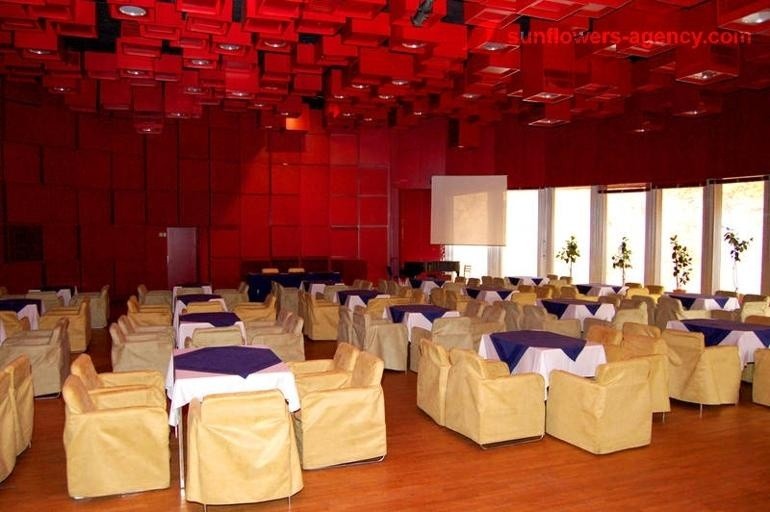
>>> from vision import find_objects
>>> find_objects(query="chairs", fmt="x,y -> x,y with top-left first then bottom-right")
0,256 -> 770,512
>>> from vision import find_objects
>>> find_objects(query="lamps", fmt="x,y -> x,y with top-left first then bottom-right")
409,0 -> 435,30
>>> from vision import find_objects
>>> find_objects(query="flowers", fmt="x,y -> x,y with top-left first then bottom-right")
667,234 -> 695,288
555,235 -> 581,275
723,226 -> 755,289
611,236 -> 633,285
